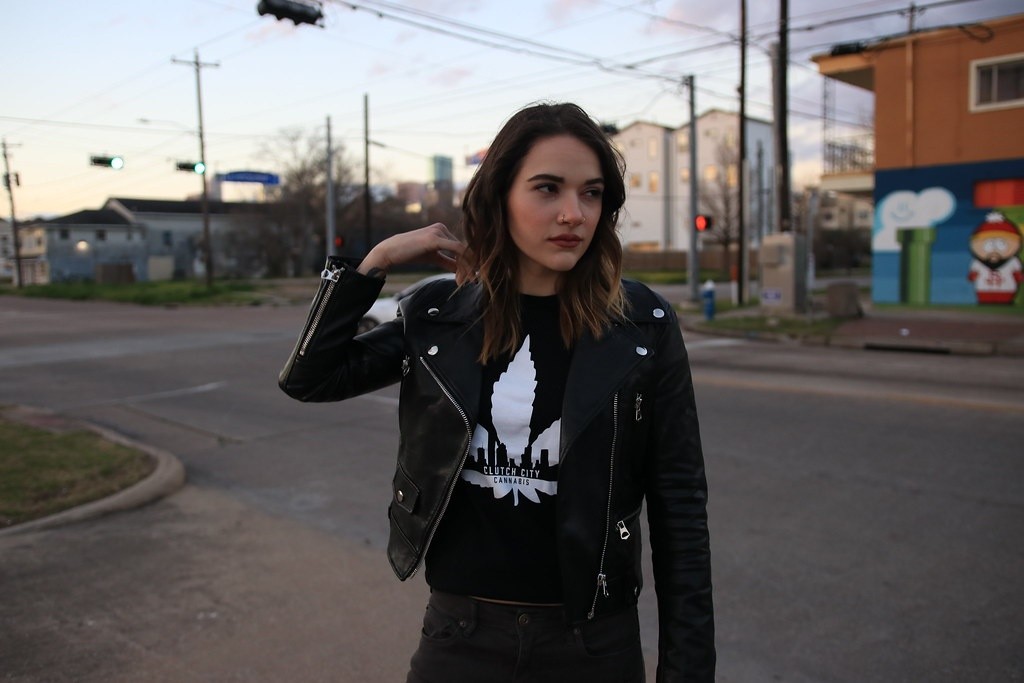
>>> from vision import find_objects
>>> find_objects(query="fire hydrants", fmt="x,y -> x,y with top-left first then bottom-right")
703,279 -> 715,321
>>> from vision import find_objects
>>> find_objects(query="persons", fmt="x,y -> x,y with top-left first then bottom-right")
278,103 -> 715,683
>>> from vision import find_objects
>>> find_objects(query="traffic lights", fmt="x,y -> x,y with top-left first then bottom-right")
93,156 -> 122,171
695,215 -> 712,231
178,163 -> 205,175
